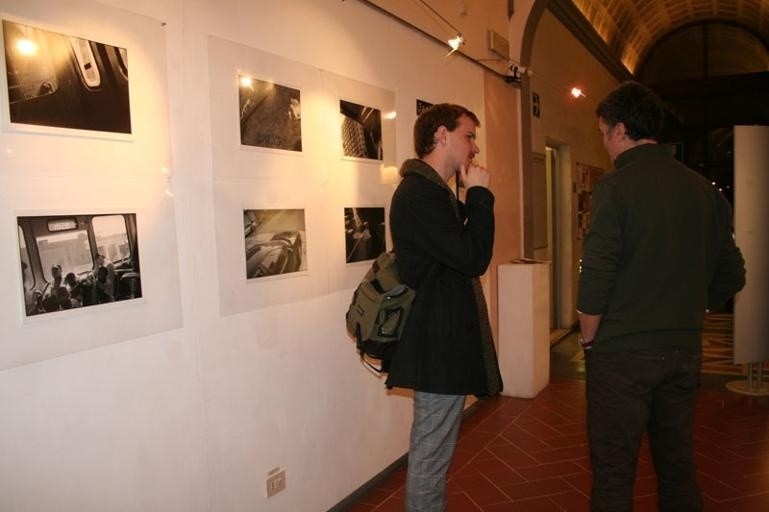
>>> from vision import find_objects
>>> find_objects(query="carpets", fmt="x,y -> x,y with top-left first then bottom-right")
569,314 -> 769,377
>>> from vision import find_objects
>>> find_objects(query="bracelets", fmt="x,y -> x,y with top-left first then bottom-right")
577,332 -> 594,347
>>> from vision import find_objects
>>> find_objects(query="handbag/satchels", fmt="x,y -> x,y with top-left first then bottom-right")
346,248 -> 416,357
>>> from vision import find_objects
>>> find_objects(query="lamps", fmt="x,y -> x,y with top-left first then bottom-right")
419,1 -> 468,59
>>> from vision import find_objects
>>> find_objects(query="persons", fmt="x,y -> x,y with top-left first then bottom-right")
390,104 -> 503,512
21,254 -> 115,316
575,80 -> 746,512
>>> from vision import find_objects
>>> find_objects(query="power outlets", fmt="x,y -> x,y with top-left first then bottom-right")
263,468 -> 288,499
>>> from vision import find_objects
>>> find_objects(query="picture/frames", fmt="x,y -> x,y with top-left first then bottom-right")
1,13 -> 136,142
236,67 -> 392,290
11,207 -> 150,327
